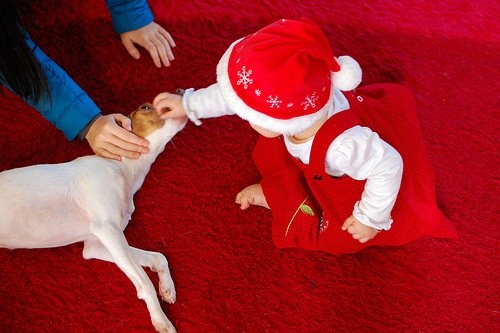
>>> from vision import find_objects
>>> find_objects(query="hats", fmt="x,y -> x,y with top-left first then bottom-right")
215,17 -> 363,135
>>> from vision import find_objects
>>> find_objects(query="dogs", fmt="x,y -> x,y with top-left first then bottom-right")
1,100 -> 190,333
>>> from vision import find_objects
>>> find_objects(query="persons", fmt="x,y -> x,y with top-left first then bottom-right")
153,18 -> 458,254
0,0 -> 175,160
